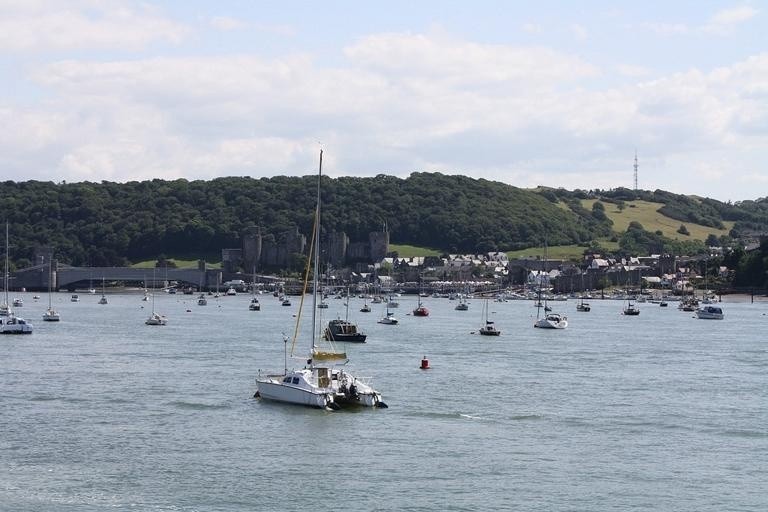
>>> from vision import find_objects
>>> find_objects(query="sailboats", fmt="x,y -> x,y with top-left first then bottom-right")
409,279 -> 430,316
0,223 -> 35,335
477,298 -> 502,336
533,255 -> 572,330
42,256 -> 60,322
254,150 -> 389,411
316,278 -> 398,313
576,269 -> 592,312
637,241 -> 724,323
98,276 -> 109,305
622,282 -> 642,315
145,269 -> 170,326
322,288 -> 368,342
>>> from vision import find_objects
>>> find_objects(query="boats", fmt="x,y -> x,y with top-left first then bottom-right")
454,297 -> 468,311
226,286 -> 236,295
197,293 -> 207,307
12,298 -> 24,307
376,311 -> 399,325
70,294 -> 79,302
248,298 -> 261,310
419,281 -> 475,300
272,280 -> 292,306
